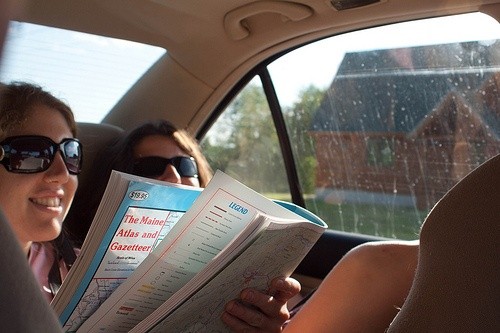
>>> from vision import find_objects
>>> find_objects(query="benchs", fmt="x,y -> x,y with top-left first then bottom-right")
61,121 -> 125,249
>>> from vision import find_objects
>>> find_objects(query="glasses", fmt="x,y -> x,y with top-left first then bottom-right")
132,154 -> 199,179
0,134 -> 84,175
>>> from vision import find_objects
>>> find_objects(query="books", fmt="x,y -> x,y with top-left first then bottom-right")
50,169 -> 328,333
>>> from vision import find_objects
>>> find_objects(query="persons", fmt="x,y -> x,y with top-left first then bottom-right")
0,82 -> 301,333
74,119 -> 420,333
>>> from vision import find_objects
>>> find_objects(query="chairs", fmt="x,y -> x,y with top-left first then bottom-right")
388,154 -> 500,333
